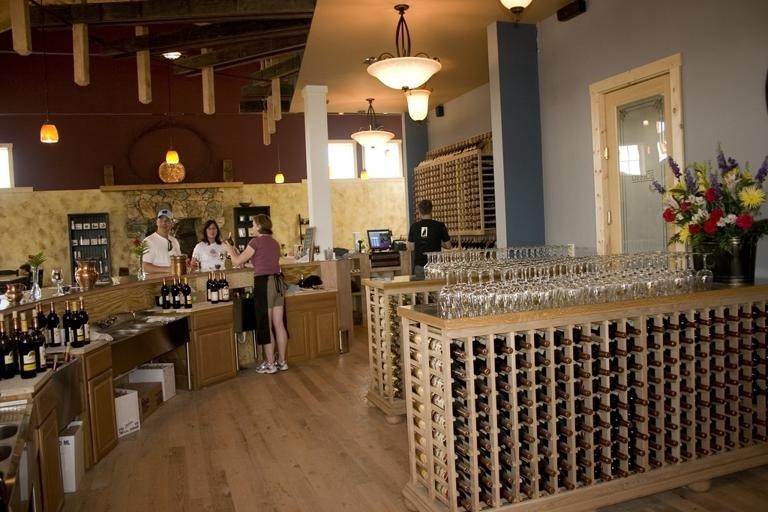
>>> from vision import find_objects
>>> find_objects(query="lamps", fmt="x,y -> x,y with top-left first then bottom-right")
363,4 -> 441,91
499,0 -> 532,16
39,71 -> 58,143
351,98 -> 396,150
359,148 -> 369,180
166,63 -> 180,164
405,82 -> 431,123
275,135 -> 284,184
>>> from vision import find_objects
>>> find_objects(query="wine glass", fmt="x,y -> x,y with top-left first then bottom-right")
51,265 -> 64,296
423,246 -> 717,320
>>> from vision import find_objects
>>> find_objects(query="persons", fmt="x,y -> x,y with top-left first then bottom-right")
190,219 -> 231,273
137,209 -> 182,279
408,200 -> 451,277
225,214 -> 288,374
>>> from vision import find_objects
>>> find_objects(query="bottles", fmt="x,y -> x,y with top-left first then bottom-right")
206,271 -> 231,305
79,236 -> 84,246
226,231 -> 234,259
155,276 -> 193,310
98,260 -> 108,274
74,260 -> 98,289
413,131 -> 495,231
97,235 -> 102,245
1,296 -> 91,379
369,290 -> 766,512
74,250 -> 81,259
450,232 -> 496,248
194,258 -> 201,272
299,273 -> 305,287
71,220 -> 75,229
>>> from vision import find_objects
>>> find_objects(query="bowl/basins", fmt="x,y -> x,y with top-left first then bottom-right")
239,202 -> 251,207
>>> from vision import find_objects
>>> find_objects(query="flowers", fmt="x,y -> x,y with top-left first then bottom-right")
132,238 -> 150,276
653,144 -> 768,254
26,253 -> 47,298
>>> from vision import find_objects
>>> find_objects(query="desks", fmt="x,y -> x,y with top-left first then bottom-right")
0,275 -> 29,297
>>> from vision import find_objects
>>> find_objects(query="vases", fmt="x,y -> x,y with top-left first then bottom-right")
690,227 -> 757,287
29,267 -> 41,299
136,255 -> 146,280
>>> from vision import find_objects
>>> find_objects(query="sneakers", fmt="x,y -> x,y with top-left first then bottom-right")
255,360 -> 278,374
275,360 -> 288,371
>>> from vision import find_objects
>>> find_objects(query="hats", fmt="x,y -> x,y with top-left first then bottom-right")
157,209 -> 171,218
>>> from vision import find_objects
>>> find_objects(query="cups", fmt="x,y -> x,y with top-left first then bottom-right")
62,285 -> 72,295
215,259 -> 221,269
5,283 -> 27,302
170,255 -> 187,275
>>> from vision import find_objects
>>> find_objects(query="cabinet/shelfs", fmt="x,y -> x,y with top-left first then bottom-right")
46,335 -> 116,470
359,251 -> 768,512
413,132 -> 497,238
279,286 -> 340,364
143,300 -> 237,390
0,368 -> 68,512
67,212 -> 112,285
234,205 -> 271,253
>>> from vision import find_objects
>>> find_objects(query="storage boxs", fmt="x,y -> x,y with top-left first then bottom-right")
128,364 -> 177,401
58,421 -> 86,491
113,386 -> 139,437
119,382 -> 163,419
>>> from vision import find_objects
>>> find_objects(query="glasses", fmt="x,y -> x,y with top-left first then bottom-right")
167,239 -> 173,251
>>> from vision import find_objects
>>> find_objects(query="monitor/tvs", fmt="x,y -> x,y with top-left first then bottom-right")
367,229 -> 391,253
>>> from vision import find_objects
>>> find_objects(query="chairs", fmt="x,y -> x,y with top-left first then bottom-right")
28,269 -> 44,290
0,269 -> 20,276
